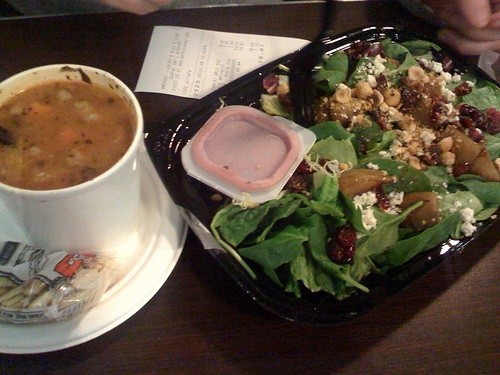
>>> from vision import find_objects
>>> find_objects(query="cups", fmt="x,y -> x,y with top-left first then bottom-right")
0,64 -> 143,259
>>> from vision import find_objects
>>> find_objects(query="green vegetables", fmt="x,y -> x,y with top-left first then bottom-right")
210,37 -> 500,299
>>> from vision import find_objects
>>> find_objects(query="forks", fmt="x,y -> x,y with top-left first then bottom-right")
287,1 -> 343,127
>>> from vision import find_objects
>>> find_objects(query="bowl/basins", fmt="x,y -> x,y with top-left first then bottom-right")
144,21 -> 500,327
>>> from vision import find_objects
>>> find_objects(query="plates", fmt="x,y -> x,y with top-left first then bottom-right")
0,144 -> 189,354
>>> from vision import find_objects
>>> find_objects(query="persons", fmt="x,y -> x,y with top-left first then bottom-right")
404,0 -> 500,68
